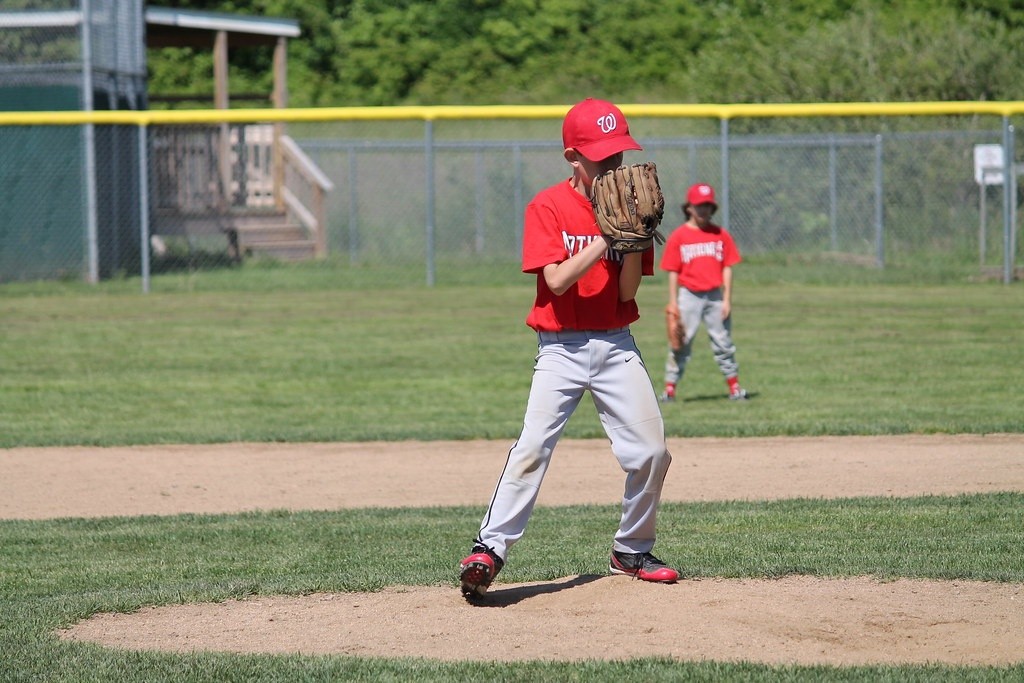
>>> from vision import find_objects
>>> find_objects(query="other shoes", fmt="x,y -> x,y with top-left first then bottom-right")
660,382 -> 676,402
728,377 -> 746,399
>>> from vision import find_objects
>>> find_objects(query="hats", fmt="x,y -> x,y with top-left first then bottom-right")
562,98 -> 643,163
688,183 -> 716,205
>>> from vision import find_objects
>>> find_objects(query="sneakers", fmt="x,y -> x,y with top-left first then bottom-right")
459,538 -> 504,602
610,549 -> 678,582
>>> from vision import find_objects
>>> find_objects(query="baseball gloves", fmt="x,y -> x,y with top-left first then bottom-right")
665,306 -> 685,351
590,160 -> 667,255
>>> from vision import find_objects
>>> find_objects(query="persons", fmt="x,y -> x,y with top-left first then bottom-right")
459,98 -> 679,603
658,183 -> 746,402
